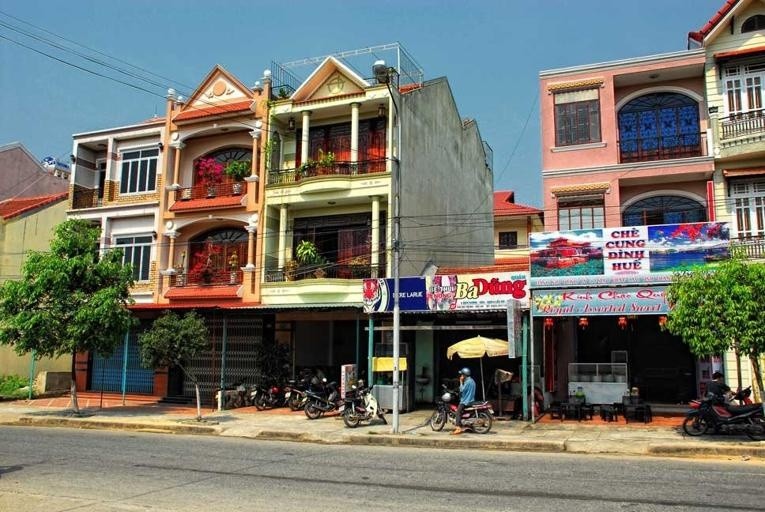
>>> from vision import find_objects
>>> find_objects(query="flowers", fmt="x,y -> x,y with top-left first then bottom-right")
190,241 -> 222,278
284,260 -> 298,272
194,156 -> 223,187
349,256 -> 370,271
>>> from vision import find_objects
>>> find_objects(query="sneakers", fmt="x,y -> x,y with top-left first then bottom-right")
454,428 -> 462,433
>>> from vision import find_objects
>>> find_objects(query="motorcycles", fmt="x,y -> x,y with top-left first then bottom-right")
425,385 -> 494,434
681,386 -> 765,441
213,363 -> 388,428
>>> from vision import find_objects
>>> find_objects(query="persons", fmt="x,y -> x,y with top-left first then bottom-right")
704,372 -> 740,432
450,366 -> 477,436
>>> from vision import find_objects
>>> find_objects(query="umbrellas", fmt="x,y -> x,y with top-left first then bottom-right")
446,334 -> 509,402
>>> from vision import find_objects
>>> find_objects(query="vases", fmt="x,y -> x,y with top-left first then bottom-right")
286,270 -> 296,281
207,188 -> 216,197
203,273 -> 212,283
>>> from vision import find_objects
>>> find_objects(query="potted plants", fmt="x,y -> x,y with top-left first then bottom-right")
224,159 -> 250,197
314,256 -> 328,278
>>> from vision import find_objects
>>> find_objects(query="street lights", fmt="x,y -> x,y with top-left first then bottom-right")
372,58 -> 404,437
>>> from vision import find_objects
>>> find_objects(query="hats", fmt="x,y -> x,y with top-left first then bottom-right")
459,368 -> 470,375
